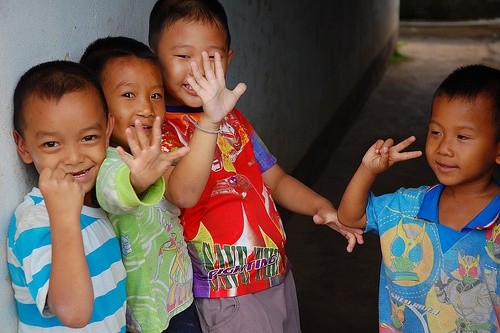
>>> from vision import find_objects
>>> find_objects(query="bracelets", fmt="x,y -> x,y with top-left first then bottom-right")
193,121 -> 223,135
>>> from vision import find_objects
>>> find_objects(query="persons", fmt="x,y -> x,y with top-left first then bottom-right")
338,63 -> 500,333
6,61 -> 126,333
147,1 -> 364,333
82,35 -> 203,332
